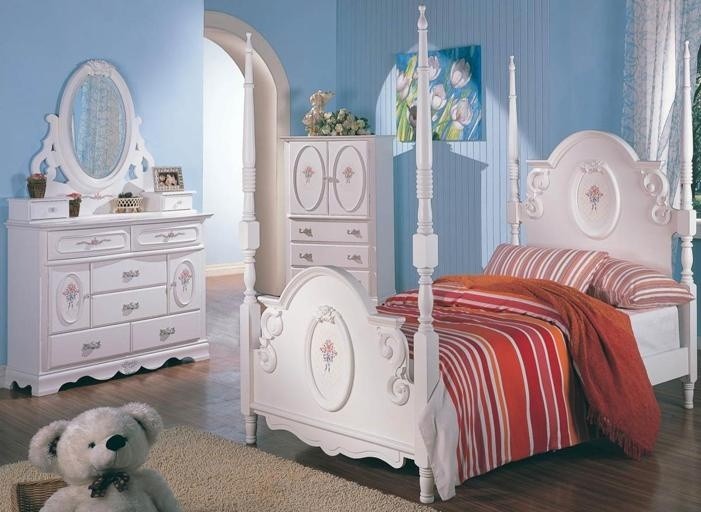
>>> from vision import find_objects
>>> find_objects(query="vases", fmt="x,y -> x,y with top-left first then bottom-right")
70,205 -> 81,218
28,180 -> 47,198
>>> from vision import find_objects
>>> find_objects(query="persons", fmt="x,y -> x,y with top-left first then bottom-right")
159,172 -> 178,186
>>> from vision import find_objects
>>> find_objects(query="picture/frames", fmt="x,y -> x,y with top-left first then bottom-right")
153,166 -> 185,192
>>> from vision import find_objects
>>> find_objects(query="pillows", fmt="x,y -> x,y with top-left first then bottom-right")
591,256 -> 695,316
479,240 -> 607,297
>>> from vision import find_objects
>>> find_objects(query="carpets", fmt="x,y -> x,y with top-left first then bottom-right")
0,423 -> 440,512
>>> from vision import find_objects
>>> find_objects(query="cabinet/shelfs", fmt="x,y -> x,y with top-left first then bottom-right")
142,191 -> 195,212
287,219 -> 396,308
281,136 -> 394,220
4,213 -> 213,397
6,197 -> 71,220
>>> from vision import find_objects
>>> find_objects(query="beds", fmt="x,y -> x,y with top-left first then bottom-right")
240,5 -> 700,502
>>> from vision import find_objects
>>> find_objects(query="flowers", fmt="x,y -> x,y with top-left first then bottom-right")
29,169 -> 47,184
65,193 -> 82,203
302,100 -> 372,135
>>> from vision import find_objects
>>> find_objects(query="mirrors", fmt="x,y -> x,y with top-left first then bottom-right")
30,59 -> 155,213
